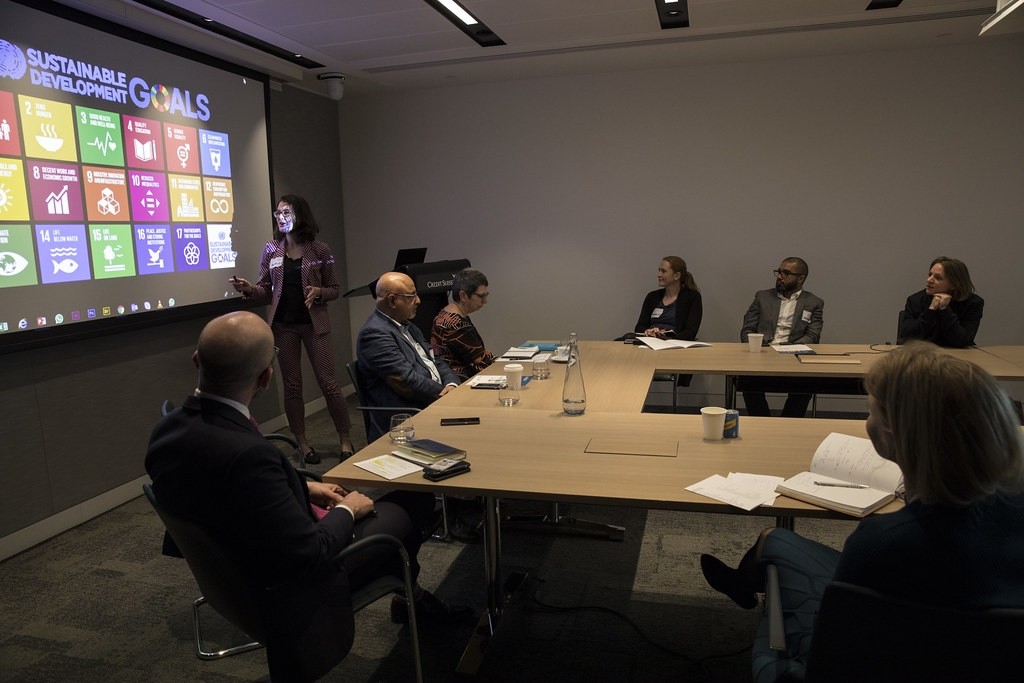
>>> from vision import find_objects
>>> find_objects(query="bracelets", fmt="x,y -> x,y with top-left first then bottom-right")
319,287 -> 322,298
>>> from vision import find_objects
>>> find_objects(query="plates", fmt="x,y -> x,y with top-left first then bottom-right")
550,356 -> 568,361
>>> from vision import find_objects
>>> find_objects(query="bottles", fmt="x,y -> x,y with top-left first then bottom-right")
562,333 -> 586,416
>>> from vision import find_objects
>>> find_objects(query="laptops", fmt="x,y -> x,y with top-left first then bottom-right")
395,248 -> 428,270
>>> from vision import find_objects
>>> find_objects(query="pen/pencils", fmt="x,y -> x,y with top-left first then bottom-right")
233,275 -> 241,294
509,358 -> 524,360
813,481 -> 869,489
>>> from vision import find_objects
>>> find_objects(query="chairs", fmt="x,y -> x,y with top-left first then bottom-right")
347,359 -> 487,541
730,336 -> 820,419
651,373 -> 678,414
895,310 -> 975,345
768,564 -> 1024,683
142,434 -> 423,683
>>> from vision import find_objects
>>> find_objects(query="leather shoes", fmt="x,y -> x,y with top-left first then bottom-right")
422,510 -> 444,544
447,515 -> 484,544
390,590 -> 475,625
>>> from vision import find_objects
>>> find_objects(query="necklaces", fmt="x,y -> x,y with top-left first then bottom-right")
453,302 -> 465,317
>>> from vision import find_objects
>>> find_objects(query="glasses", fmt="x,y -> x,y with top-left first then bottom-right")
774,270 -> 806,279
385,290 -> 417,298
274,208 -> 292,219
467,292 -> 489,298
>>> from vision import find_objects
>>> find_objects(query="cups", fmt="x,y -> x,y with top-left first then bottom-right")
504,363 -> 523,391
499,380 -> 519,407
389,413 -> 415,443
532,360 -> 551,378
554,347 -> 570,357
747,333 -> 764,353
700,406 -> 727,439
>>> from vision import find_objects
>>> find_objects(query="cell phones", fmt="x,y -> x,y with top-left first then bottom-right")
441,418 -> 480,425
471,383 -> 507,389
423,461 -> 472,482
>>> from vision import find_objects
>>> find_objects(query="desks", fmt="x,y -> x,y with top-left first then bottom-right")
322,340 -> 1024,675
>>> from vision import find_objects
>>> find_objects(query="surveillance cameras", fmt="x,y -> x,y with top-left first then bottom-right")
325,77 -> 344,101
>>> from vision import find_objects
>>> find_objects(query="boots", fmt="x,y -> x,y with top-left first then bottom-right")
701,533 -> 762,609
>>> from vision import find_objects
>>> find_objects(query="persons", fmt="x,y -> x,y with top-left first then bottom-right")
228,194 -> 355,464
145,310 -> 451,683
900,256 -> 984,349
356,272 -> 508,543
612,255 -> 702,386
740,257 -> 824,418
431,267 -> 497,382
699,339 -> 1023,683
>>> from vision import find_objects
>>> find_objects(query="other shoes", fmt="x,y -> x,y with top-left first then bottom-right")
340,444 -> 355,463
302,445 -> 321,465
